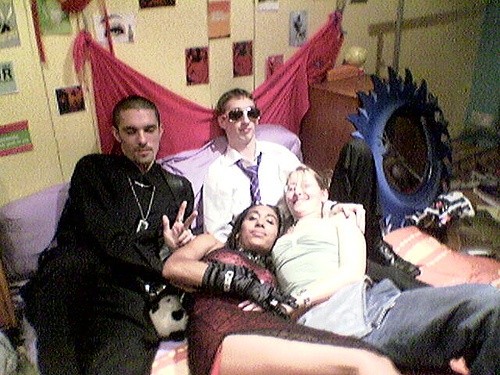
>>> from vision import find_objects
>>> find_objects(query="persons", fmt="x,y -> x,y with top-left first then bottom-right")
160,202 -> 401,375
19,92 -> 199,375
202,88 -> 434,293
271,166 -> 500,375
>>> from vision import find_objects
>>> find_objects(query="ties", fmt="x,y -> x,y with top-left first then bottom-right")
235,152 -> 263,206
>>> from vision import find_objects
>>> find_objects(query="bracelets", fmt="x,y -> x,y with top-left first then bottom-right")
330,202 -> 339,211
298,291 -> 313,312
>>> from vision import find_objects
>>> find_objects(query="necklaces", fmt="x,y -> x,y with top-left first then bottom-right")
123,174 -> 158,233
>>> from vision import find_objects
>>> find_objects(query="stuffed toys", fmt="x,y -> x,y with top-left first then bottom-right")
149,285 -> 189,343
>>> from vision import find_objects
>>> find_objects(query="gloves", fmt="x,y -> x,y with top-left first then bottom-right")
201,261 -> 299,321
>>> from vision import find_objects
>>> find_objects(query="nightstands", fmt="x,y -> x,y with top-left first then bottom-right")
300,72 -> 418,191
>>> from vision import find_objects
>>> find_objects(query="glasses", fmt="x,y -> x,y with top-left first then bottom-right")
222,107 -> 261,121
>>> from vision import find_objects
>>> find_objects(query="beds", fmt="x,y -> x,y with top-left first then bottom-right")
0,124 -> 500,375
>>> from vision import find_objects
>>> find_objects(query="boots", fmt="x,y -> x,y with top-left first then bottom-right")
364,220 -> 420,278
